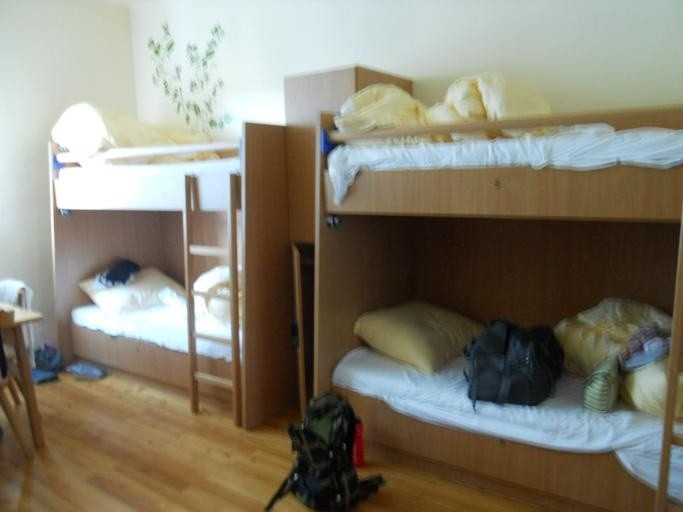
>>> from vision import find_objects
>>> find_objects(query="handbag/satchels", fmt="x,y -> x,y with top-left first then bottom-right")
582,352 -> 621,414
460,313 -> 566,411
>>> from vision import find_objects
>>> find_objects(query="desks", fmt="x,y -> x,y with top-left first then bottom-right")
0,304 -> 46,460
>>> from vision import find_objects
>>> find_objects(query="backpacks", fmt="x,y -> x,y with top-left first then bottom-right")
263,389 -> 384,512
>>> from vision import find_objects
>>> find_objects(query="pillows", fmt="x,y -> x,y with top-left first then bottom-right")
357,301 -> 485,375
80,267 -> 185,318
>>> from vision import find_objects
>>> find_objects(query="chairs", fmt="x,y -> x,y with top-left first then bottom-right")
0,277 -> 36,404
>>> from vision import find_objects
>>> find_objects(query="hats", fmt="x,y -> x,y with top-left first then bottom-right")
618,321 -> 671,371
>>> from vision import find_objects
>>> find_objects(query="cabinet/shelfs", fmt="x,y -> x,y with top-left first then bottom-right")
283,65 -> 415,240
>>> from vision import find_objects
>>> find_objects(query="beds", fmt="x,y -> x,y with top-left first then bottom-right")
315,111 -> 683,511
48,122 -> 287,432
